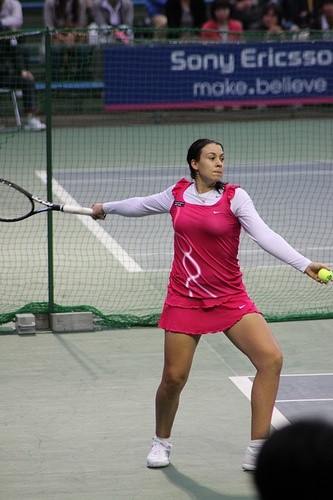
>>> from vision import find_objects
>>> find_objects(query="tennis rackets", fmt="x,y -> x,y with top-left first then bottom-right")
0,179 -> 101,223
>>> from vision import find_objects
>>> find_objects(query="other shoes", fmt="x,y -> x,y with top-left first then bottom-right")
28,118 -> 46,129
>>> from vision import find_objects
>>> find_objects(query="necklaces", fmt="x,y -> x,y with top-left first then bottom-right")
195,185 -> 212,205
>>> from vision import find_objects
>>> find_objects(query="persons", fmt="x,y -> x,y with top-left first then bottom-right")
251,415 -> 333,500
90,139 -> 333,473
0,0 -> 333,139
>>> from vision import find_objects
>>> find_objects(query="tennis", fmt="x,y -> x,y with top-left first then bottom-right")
318,268 -> 332,283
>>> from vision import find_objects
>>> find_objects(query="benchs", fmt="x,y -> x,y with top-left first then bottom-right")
19,0 -> 110,94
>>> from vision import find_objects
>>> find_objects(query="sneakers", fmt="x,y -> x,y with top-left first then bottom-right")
242,442 -> 263,470
146,437 -> 176,467
